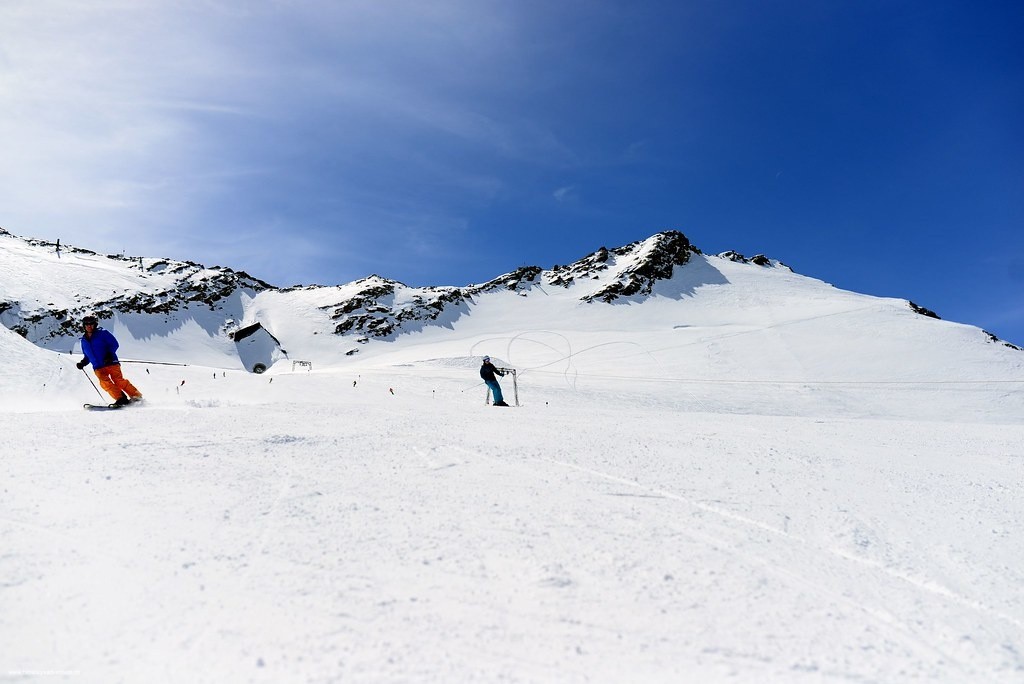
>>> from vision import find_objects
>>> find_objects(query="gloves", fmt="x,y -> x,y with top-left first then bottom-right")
77,361 -> 86,369
104,355 -> 113,364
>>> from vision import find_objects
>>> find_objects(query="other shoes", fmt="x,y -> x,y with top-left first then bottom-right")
114,396 -> 140,405
494,402 -> 506,407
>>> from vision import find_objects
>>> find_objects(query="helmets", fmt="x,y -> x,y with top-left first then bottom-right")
82,317 -> 97,323
482,356 -> 489,360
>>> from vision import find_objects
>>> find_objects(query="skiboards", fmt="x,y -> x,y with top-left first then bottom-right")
493,402 -> 526,408
82,399 -> 149,412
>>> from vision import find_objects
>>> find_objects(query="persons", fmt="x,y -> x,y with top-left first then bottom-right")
480,355 -> 508,406
75,316 -> 143,407
